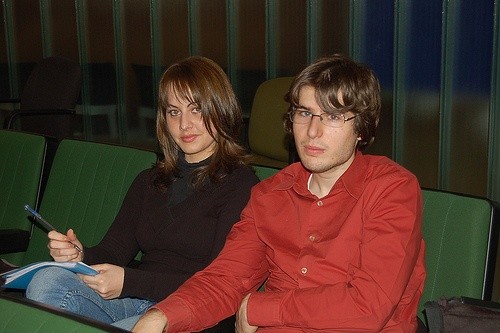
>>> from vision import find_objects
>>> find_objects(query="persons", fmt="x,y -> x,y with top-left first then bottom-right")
129,55 -> 427,333
26,55 -> 261,332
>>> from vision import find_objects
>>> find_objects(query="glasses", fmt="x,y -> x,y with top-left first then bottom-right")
287,106 -> 360,130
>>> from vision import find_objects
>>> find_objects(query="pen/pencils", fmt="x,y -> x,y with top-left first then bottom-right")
24,204 -> 85,253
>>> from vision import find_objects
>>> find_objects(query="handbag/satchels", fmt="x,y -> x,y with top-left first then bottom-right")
425,293 -> 500,333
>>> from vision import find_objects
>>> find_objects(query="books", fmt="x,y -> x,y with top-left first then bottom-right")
0,260 -> 100,289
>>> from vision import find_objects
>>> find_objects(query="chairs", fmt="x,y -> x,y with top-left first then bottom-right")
0,56 -> 81,170
418,187 -> 500,325
0,130 -> 44,264
20,139 -> 162,268
237,77 -> 298,168
0,292 -> 117,333
